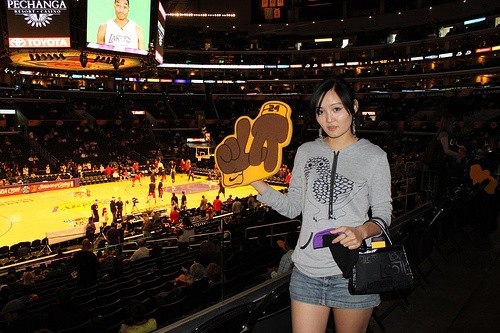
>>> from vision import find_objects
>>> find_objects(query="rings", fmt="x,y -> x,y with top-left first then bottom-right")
343,232 -> 348,238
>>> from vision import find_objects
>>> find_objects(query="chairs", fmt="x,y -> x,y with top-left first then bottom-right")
0,163 -> 491,333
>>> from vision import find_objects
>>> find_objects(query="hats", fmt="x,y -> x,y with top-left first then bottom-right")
314,228 -> 358,278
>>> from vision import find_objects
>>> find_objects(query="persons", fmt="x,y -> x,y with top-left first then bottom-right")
1,48 -> 500,333
250,77 -> 393,333
97,0 -> 144,50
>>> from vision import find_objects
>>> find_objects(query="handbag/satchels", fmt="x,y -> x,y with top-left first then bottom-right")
348,217 -> 415,295
